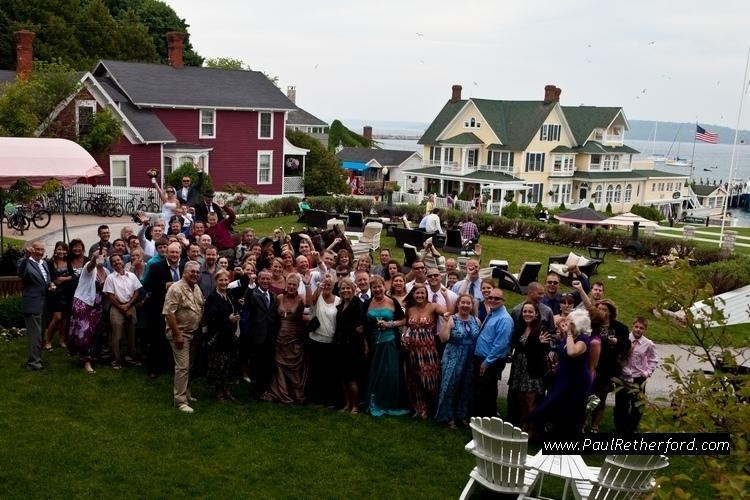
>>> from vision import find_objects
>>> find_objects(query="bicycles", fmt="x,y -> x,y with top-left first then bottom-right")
10,204 -> 51,231
146,187 -> 160,214
125,193 -> 149,216
13,204 -> 25,236
80,192 -> 124,218
47,191 -> 80,216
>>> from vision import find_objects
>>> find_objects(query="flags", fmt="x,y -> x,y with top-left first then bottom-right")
694,124 -> 719,145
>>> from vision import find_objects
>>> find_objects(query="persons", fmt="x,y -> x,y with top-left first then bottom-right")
162,259 -> 206,413
332,276 -> 370,417
447,195 -> 455,209
452,258 -> 487,303
200,266 -> 250,405
434,293 -> 483,427
16,240 -> 57,370
369,248 -> 391,276
506,281 -> 556,364
311,249 -> 338,281
383,259 -> 413,290
148,177 -> 180,234
541,265 -> 591,313
384,272 -> 410,349
531,310 -> 592,440
505,302 -> 551,427
349,253 -> 373,282
574,279 -> 631,435
401,283 -> 452,419
418,210 -> 433,231
577,282 -> 604,309
468,287 -> 515,418
88,204 -> 296,294
425,208 -> 445,235
439,244 -> 482,290
245,270 -> 278,370
140,243 -> 188,378
612,317 -> 658,436
68,239 -> 89,300
333,265 -> 352,297
175,176 -> 199,205
66,248 -> 111,375
101,254 -> 145,371
353,270 -> 373,311
260,270 -> 314,404
308,276 -> 343,410
548,293 -> 576,373
43,241 -> 68,352
301,197 -> 312,211
423,268 -> 460,358
294,250 -> 328,292
298,238 -> 314,255
336,249 -> 351,265
459,265 -> 496,323
404,260 -> 431,295
474,195 -> 482,215
195,190 -> 223,227
420,236 -> 444,272
460,215 -> 480,239
425,193 -> 435,214
441,258 -> 465,286
444,272 -> 459,290
357,273 -> 411,418
588,304 -> 601,387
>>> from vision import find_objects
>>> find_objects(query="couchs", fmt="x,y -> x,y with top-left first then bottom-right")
548,254 -> 596,286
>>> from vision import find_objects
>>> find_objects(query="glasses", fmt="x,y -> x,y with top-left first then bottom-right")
488,295 -> 505,301
35,249 -> 46,252
547,281 -> 559,285
207,195 -> 214,198
102,231 -> 111,235
183,180 -> 190,183
166,191 -> 173,193
414,267 -> 425,270
337,273 -> 349,278
426,274 -> 439,278
561,293 -> 573,297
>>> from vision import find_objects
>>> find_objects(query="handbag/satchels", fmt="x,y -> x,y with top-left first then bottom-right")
307,317 -> 320,332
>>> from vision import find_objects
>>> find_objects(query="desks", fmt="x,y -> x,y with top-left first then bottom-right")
589,247 -> 608,264
525,446 -> 594,500
385,223 -> 399,236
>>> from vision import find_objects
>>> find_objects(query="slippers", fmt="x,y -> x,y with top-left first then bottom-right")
127,359 -> 142,367
88,370 -> 97,375
112,364 -> 122,370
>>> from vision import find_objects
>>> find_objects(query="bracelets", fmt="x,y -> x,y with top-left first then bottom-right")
464,277 -> 472,283
304,284 -> 311,288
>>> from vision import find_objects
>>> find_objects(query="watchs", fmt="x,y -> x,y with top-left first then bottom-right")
317,261 -> 323,265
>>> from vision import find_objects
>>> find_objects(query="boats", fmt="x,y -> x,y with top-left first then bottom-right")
704,168 -> 711,171
710,166 -> 717,168
646,153 -> 668,161
666,156 -> 695,166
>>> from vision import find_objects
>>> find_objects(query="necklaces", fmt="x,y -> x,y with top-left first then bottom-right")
371,297 -> 386,304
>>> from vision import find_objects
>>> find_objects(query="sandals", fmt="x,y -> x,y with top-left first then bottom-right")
350,410 -> 361,414
590,428 -> 599,434
45,347 -> 53,352
59,343 -> 66,348
338,408 -> 348,412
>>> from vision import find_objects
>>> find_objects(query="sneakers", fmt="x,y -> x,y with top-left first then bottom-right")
189,397 -> 197,401
178,404 -> 194,414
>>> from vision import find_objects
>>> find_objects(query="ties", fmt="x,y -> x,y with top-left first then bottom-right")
632,340 -> 639,350
433,295 -> 438,334
170,267 -> 179,282
469,282 -> 475,296
361,294 -> 369,300
473,313 -> 492,354
263,293 -> 270,308
154,248 -> 157,256
207,204 -> 211,211
212,228 -> 216,246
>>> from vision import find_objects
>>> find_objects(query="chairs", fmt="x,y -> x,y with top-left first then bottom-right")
505,262 -> 541,291
394,227 -> 437,250
403,216 -> 420,232
458,417 -> 540,500
328,218 -> 345,235
445,231 -> 473,254
562,454 -> 668,500
360,222 -> 382,252
489,260 -> 508,287
297,203 -> 316,222
348,211 -> 364,227
